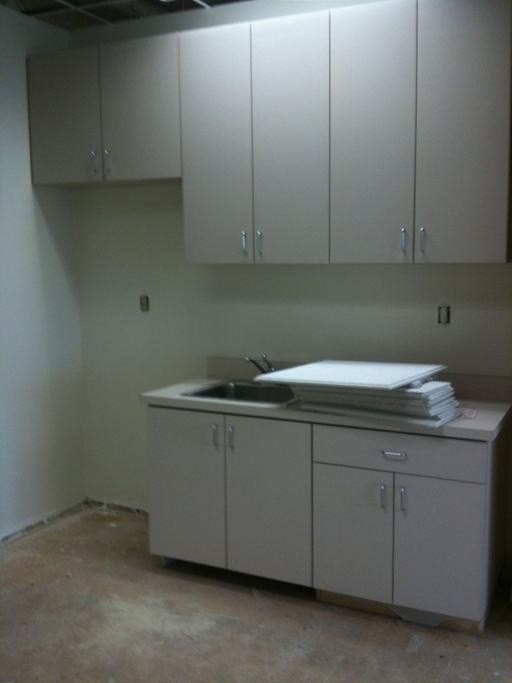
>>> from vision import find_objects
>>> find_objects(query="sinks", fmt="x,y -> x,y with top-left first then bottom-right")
179,379 -> 294,405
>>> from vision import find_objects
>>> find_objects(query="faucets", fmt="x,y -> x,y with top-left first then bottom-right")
243,353 -> 274,374
